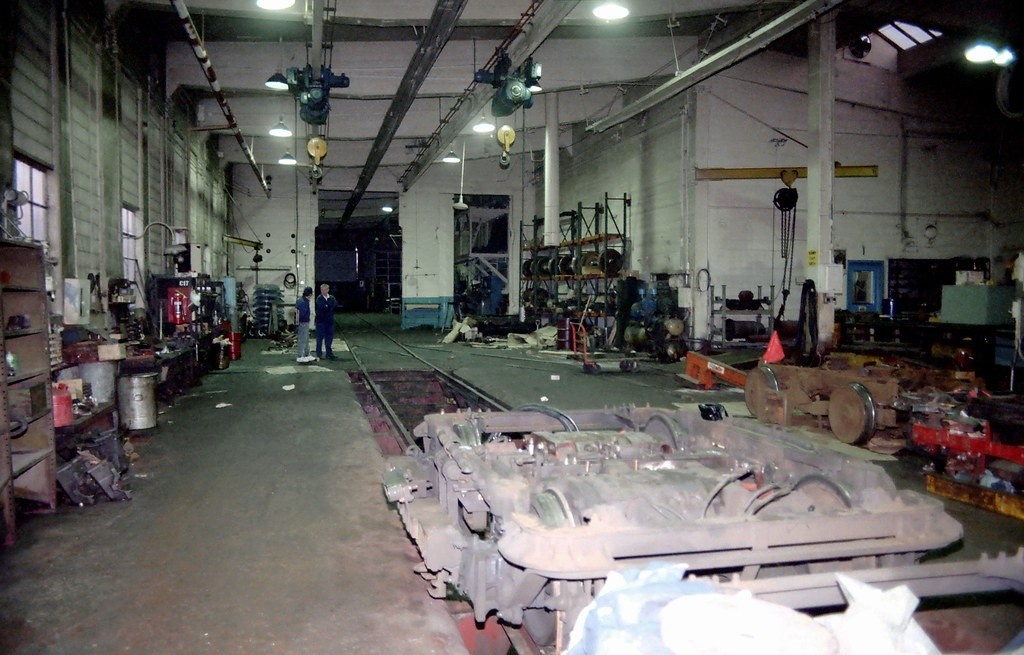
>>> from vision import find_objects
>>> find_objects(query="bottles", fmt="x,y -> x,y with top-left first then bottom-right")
638,288 -> 658,313
889,298 -> 896,320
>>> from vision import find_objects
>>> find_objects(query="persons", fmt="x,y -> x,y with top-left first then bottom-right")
314,284 -> 337,361
296,286 -> 315,362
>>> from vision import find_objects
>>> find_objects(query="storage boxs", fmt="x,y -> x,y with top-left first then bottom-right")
941,284 -> 1013,325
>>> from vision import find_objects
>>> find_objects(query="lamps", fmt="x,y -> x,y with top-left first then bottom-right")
443,141 -> 460,164
264,35 -> 298,166
473,108 -> 495,135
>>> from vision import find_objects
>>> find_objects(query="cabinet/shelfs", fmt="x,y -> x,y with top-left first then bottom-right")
518,193 -> 631,344
0,238 -> 58,547
709,283 -> 775,350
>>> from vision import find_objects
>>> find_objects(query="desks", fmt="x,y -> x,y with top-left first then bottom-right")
56,401 -> 118,442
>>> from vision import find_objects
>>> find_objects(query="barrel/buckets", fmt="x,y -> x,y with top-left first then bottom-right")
120,372 -> 159,429
227,329 -> 242,361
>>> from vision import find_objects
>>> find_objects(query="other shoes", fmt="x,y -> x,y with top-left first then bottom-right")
317,354 -> 322,359
305,355 -> 317,362
296,357 -> 309,363
326,354 -> 336,361
